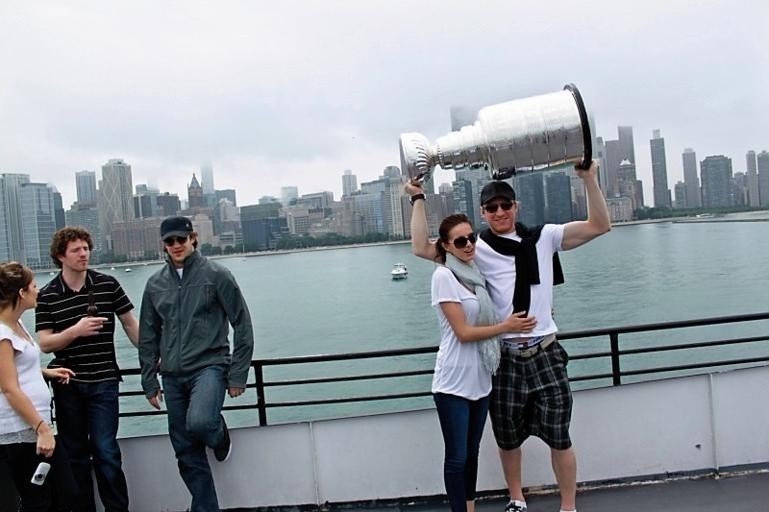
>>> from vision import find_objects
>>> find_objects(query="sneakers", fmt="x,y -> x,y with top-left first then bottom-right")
213,413 -> 231,463
504,503 -> 527,512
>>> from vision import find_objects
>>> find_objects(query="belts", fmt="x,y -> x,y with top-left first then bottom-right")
500,334 -> 555,358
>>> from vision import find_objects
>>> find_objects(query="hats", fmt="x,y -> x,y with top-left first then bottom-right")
161,216 -> 192,241
481,181 -> 515,204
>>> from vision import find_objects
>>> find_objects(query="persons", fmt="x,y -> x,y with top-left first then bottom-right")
35,227 -> 160,512
138,216 -> 253,512
0,262 -> 77,511
405,157 -> 611,511
430,213 -> 539,512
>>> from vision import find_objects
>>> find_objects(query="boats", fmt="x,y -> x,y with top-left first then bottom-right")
391,263 -> 408,281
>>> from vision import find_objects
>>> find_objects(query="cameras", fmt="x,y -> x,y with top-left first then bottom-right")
30,461 -> 53,488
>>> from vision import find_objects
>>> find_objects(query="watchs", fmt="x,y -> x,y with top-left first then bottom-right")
408,193 -> 427,205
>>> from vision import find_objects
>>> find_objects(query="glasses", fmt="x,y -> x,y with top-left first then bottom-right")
165,237 -> 187,246
483,203 -> 513,213
448,232 -> 477,250
